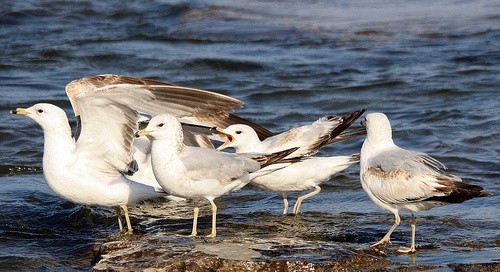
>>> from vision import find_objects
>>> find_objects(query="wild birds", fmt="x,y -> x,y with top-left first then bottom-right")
357,111 -> 494,254
9,73 -> 275,236
133,112 -> 301,238
209,123 -> 361,213
